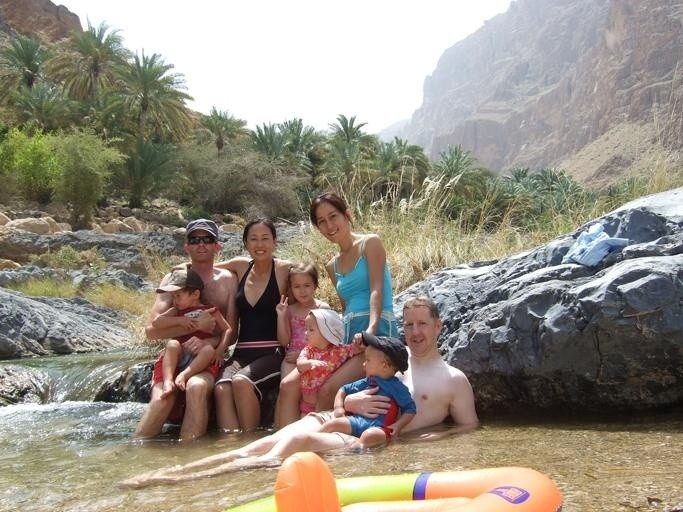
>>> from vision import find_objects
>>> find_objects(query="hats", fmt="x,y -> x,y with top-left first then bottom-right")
362,330 -> 408,375
308,308 -> 344,346
186,219 -> 218,237
156,270 -> 204,293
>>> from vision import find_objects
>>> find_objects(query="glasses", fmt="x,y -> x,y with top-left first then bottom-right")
188,237 -> 215,244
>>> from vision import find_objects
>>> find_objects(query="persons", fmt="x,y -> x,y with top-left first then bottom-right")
170,216 -> 292,430
278,193 -> 394,428
135,218 -> 239,441
152,270 -> 232,399
296,309 -> 363,420
117,297 -> 479,488
273,263 -> 331,426
316,331 -> 416,449
